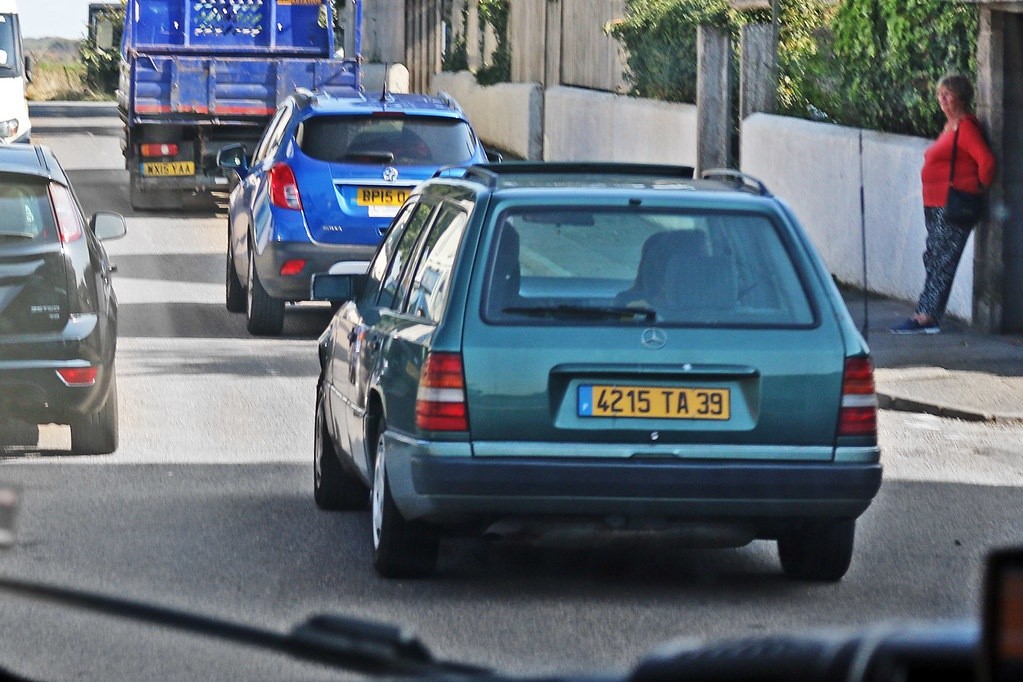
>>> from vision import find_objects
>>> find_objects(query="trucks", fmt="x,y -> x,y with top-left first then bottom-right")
0,0 -> 36,143
112,0 -> 366,212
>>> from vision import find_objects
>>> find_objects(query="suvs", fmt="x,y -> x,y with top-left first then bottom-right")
216,83 -> 503,339
0,142 -> 128,459
304,159 -> 889,586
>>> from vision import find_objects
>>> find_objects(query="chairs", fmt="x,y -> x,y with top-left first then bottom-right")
651,256 -> 737,314
616,230 -> 712,311
494,225 -> 521,309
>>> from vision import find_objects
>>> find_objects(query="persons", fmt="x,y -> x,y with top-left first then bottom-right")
889,75 -> 995,334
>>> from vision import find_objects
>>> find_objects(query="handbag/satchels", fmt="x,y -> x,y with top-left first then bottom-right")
947,187 -> 982,224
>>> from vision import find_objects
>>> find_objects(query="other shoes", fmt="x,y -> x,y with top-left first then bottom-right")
889,318 -> 941,336
